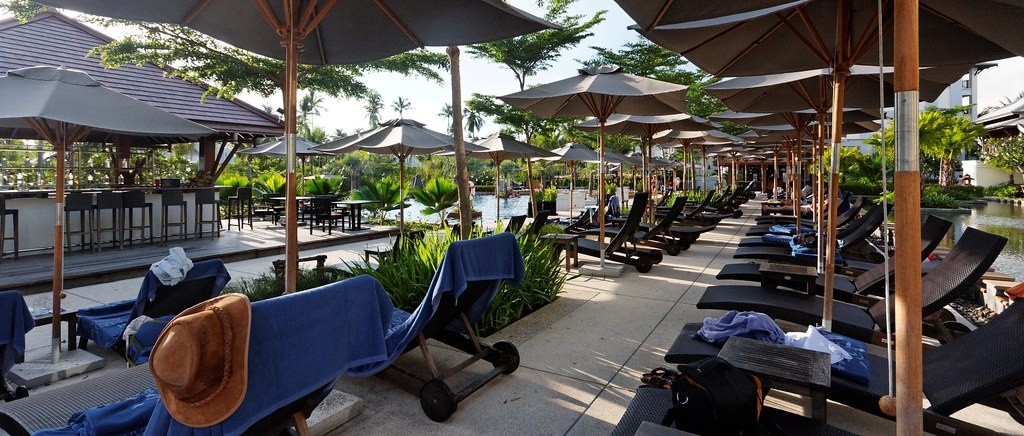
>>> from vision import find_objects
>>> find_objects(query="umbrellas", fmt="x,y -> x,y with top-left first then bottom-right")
525,142 -> 625,225
235,136 -> 336,198
32,1 -> 563,295
572,108 -> 719,226
0,64 -> 219,364
581,147 -> 681,209
307,119 -> 490,232
435,134 -> 565,223
615,0 -> 1024,436
496,64 -> 690,267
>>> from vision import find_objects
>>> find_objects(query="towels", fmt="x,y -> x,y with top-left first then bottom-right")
608,195 -> 621,219
74,257 -> 232,351
690,304 -> 786,347
66,385 -> 162,436
341,232 -> 525,379
30,273 -> 395,436
0,289 -> 35,378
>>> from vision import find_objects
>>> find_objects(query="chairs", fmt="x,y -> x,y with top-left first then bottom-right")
228,187 -> 253,230
256,196 -> 350,235
0,258 -> 392,435
504,180 -> 1024,436
195,187 -> 221,238
123,190 -> 154,247
161,189 -> 187,242
0,194 -> 19,263
93,192 -> 124,252
347,232 -> 519,422
65,189 -> 94,256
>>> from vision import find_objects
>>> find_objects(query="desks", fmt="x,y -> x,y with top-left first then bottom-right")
758,261 -> 819,296
331,199 -> 380,231
270,196 -> 315,227
541,233 -> 579,272
33,303 -> 78,352
714,334 -> 830,422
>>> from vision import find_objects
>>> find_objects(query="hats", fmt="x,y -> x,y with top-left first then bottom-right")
149,292 -> 251,426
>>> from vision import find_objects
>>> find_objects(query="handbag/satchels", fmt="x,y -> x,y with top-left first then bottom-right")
672,357 -> 765,436
